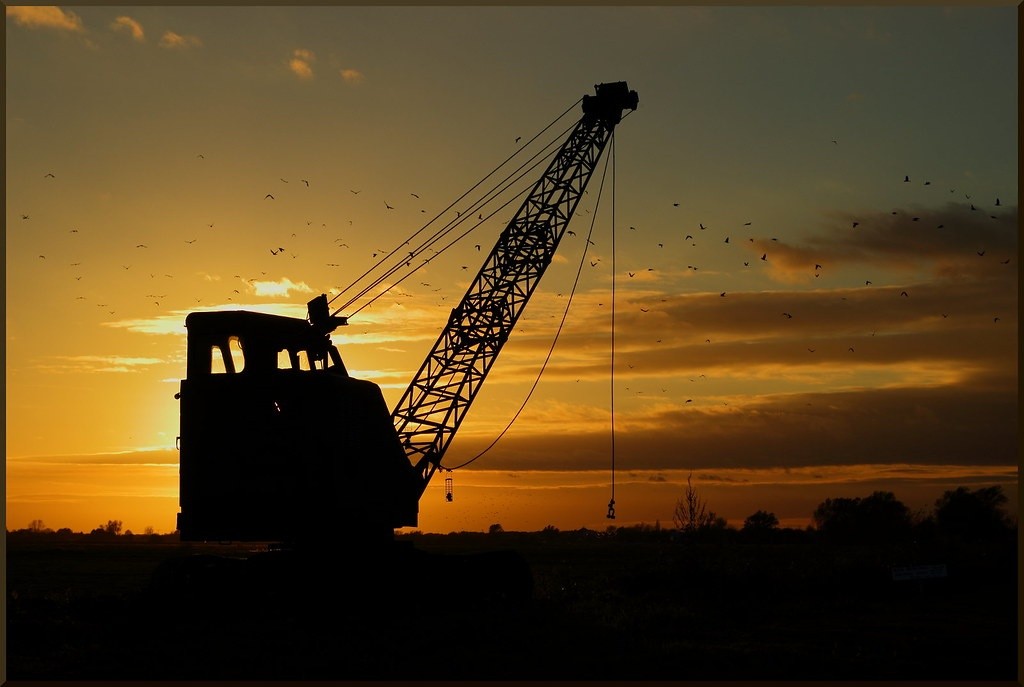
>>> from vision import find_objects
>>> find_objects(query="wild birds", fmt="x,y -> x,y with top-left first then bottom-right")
22,131 -> 1024,411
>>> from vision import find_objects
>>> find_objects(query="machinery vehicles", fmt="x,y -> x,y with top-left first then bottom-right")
175,81 -> 641,542
